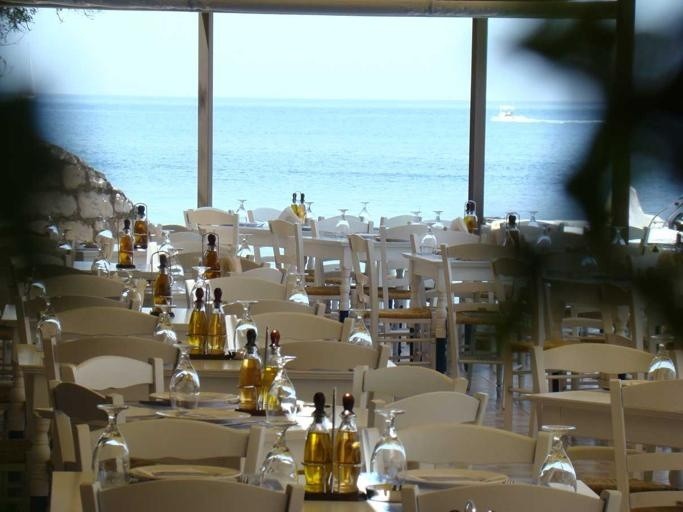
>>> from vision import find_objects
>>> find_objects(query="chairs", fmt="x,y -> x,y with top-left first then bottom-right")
34,305 -> 174,350
246,208 -> 316,277
618,225 -> 650,262
396,423 -> 552,485
346,233 -> 435,366
268,219 -> 364,323
12,276 -> 143,301
205,300 -> 329,321
40,335 -> 180,398
72,421 -> 268,480
204,275 -> 296,304
364,213 -> 426,337
317,214 -> 375,312
15,293 -> 137,344
531,343 -> 661,490
165,230 -> 208,251
352,363 -> 470,410
534,266 -> 645,348
609,376 -> 682,511
438,242 -> 573,431
400,483 -> 622,511
182,207 -> 268,279
368,391 -> 490,439
51,355 -> 166,410
225,313 -> 355,353
77,480 -> 306,512
249,337 -> 392,374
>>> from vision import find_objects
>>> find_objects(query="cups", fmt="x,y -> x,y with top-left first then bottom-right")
335,207 -> 351,239
90,403 -> 133,491
232,300 -> 260,354
256,421 -> 300,491
237,233 -> 255,263
150,304 -> 180,345
610,226 -> 626,245
168,343 -> 202,416
91,242 -> 111,279
56,228 -> 74,250
120,268 -> 142,312
418,221 -> 438,256
431,210 -> 445,230
264,354 -> 299,425
526,210 -> 540,228
646,333 -> 678,383
305,201 -> 315,219
359,201 -> 371,224
410,210 -> 422,224
286,272 -> 310,305
536,223 -> 551,248
188,266 -> 213,309
367,406 -> 410,503
34,293 -> 63,352
237,198 -> 249,223
538,423 -> 579,494
167,247 -> 186,296
346,308 -> 375,347
157,229 -> 176,255
44,214 -> 60,234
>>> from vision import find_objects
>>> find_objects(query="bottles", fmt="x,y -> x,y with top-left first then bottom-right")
261,329 -> 285,411
291,193 -> 297,215
464,203 -> 477,231
134,206 -> 148,248
207,288 -> 226,358
240,330 -> 261,411
304,392 -> 334,493
120,220 -> 132,266
298,194 -> 306,227
333,393 -> 361,491
204,234 -> 220,279
153,255 -> 171,315
188,288 -> 208,358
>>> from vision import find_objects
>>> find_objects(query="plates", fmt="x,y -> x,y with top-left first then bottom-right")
128,463 -> 246,483
395,468 -> 508,488
154,408 -> 253,424
149,391 -> 241,406
